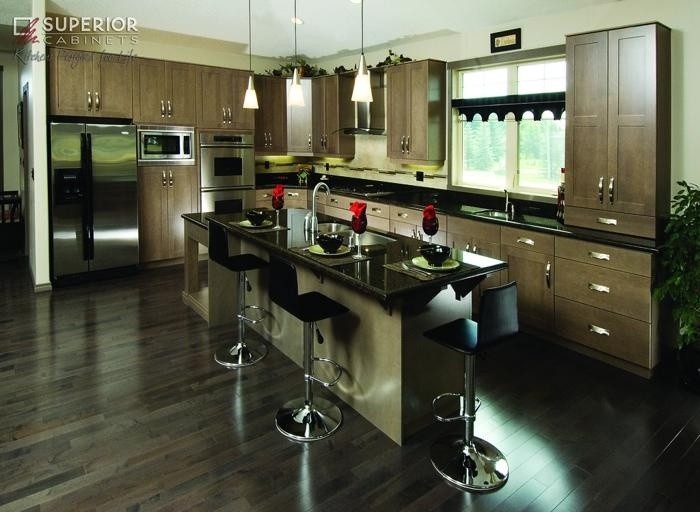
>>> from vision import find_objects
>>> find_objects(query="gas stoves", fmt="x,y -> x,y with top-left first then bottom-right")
328,183 -> 397,198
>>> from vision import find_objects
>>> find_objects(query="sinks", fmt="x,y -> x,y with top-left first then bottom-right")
475,210 -> 510,219
328,229 -> 398,247
318,220 -> 354,235
461,204 -> 492,213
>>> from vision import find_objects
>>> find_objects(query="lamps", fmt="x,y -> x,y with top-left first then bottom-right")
283,0 -> 305,107
240,0 -> 261,112
348,0 -> 376,104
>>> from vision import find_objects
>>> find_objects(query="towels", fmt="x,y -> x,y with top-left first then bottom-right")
271,183 -> 284,210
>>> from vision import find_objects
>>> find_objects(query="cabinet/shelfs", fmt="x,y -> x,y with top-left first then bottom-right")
137,162 -> 195,269
197,63 -> 252,131
501,225 -> 553,341
313,73 -> 356,159
287,78 -> 314,156
387,58 -> 447,166
563,21 -> 673,238
554,233 -> 666,379
255,73 -> 287,156
133,56 -> 198,126
46,46 -> 133,118
253,190 -> 501,300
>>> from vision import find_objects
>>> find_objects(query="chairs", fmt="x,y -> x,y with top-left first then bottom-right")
208,224 -> 268,374
420,280 -> 526,493
263,253 -> 354,441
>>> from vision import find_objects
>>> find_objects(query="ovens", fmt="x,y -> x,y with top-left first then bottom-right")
200,130 -> 256,214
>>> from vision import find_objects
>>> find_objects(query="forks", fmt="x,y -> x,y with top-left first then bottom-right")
402,262 -> 434,276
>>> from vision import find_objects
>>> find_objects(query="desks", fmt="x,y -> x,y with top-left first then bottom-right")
181,206 -> 270,321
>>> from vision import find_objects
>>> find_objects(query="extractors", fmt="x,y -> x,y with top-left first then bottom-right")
329,70 -> 387,138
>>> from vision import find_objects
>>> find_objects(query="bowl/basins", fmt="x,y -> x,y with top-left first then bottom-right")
315,232 -> 345,253
420,244 -> 452,267
246,210 -> 268,225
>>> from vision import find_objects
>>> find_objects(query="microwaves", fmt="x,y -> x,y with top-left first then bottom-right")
137,124 -> 197,166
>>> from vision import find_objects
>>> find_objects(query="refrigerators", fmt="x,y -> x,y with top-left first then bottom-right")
47,122 -> 140,281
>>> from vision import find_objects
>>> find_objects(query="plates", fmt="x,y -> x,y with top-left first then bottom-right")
239,220 -> 274,229
307,244 -> 350,256
411,255 -> 461,270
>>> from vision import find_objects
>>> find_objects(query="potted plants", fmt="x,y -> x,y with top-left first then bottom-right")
660,179 -> 700,388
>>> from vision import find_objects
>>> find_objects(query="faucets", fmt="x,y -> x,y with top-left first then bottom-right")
311,182 -> 331,233
504,189 -> 512,212
